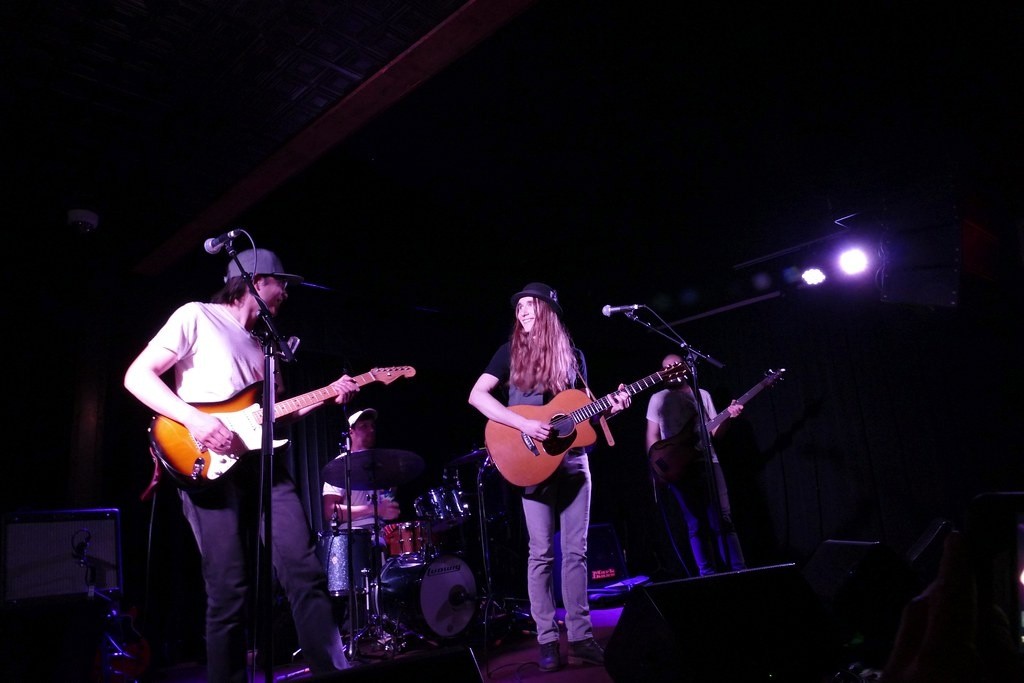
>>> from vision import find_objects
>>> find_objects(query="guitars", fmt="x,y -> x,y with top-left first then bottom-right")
145,365 -> 417,494
91,492 -> 154,682
648,366 -> 787,483
483,359 -> 692,487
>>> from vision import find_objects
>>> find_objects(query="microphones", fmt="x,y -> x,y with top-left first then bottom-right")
602,304 -> 645,317
204,229 -> 241,254
75,536 -> 92,554
454,590 -> 466,603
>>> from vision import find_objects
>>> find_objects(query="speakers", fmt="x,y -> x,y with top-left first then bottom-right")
0,508 -> 125,606
552,491 -> 1024,683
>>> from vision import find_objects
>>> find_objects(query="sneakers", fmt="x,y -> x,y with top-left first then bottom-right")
539,642 -> 560,673
567,637 -> 604,666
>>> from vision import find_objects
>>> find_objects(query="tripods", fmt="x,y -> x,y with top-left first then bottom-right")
341,466 -> 439,653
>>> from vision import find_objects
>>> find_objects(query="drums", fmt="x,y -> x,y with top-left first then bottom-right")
317,527 -> 374,599
372,553 -> 480,640
381,519 -> 433,569
412,484 -> 466,533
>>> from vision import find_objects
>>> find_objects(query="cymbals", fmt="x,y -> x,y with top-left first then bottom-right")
321,447 -> 425,491
447,450 -> 488,468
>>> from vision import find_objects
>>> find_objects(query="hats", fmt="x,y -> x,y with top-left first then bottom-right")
227,248 -> 301,282
511,282 -> 563,320
343,396 -> 378,427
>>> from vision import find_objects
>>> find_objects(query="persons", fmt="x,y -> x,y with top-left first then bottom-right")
646,354 -> 749,582
468,281 -> 632,673
123,249 -> 360,683
883,524 -> 1024,683
950,193 -> 1024,490
322,405 -> 401,643
